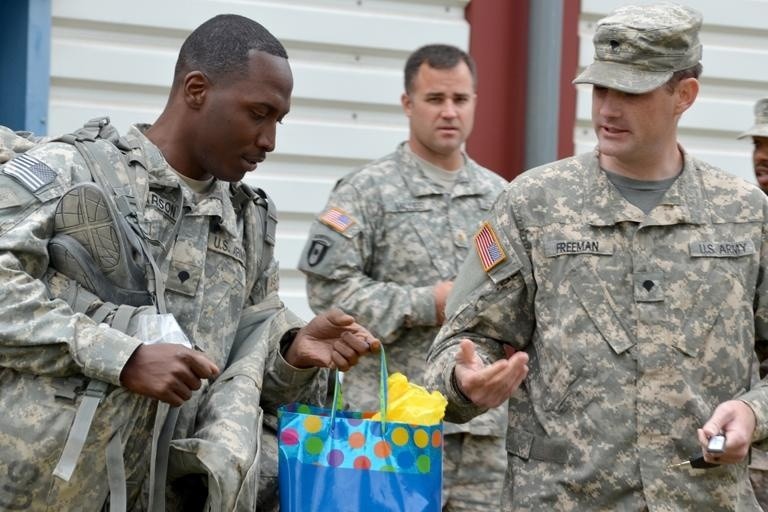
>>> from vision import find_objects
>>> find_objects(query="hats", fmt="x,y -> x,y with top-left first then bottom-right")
572,1 -> 703,95
738,98 -> 767,139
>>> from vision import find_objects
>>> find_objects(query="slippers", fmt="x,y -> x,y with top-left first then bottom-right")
49,233 -> 151,304
55,181 -> 147,290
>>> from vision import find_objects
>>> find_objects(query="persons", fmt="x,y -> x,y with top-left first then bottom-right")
297,43 -> 512,512
425,3 -> 767,512
736,97 -> 768,197
0,13 -> 383,511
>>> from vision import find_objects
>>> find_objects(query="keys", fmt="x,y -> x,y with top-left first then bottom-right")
668,454 -> 721,471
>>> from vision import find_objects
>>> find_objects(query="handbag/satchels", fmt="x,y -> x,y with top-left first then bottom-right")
0,117 -> 164,512
277,345 -> 445,512
